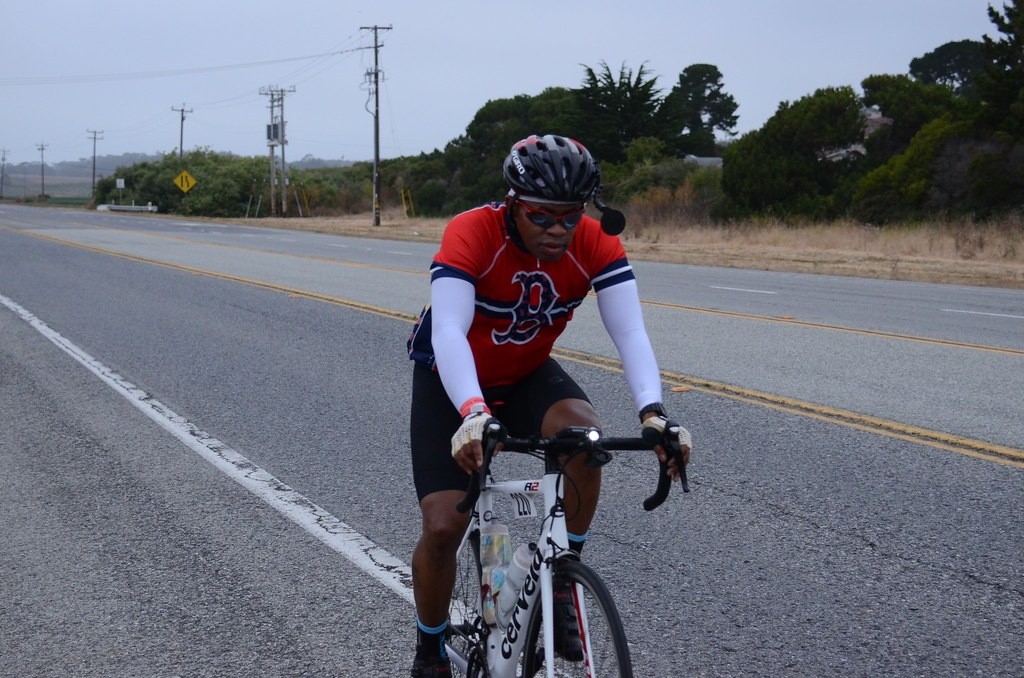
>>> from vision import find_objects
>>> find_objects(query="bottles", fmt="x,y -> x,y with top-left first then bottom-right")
479,516 -> 512,624
493,543 -> 539,630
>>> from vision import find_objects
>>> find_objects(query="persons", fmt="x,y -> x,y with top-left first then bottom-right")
406,134 -> 693,678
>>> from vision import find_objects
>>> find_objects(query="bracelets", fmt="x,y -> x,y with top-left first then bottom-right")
460,404 -> 492,420
460,396 -> 485,414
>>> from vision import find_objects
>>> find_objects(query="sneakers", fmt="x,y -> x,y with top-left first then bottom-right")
553,585 -> 585,664
409,648 -> 454,678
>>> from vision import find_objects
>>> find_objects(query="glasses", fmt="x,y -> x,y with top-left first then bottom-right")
516,200 -> 586,231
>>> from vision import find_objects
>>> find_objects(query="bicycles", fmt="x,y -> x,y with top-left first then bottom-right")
445,416 -> 691,678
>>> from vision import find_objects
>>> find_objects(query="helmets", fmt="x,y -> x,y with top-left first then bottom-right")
502,135 -> 600,204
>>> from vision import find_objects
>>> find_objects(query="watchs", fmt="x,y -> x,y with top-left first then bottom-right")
638,402 -> 670,424
